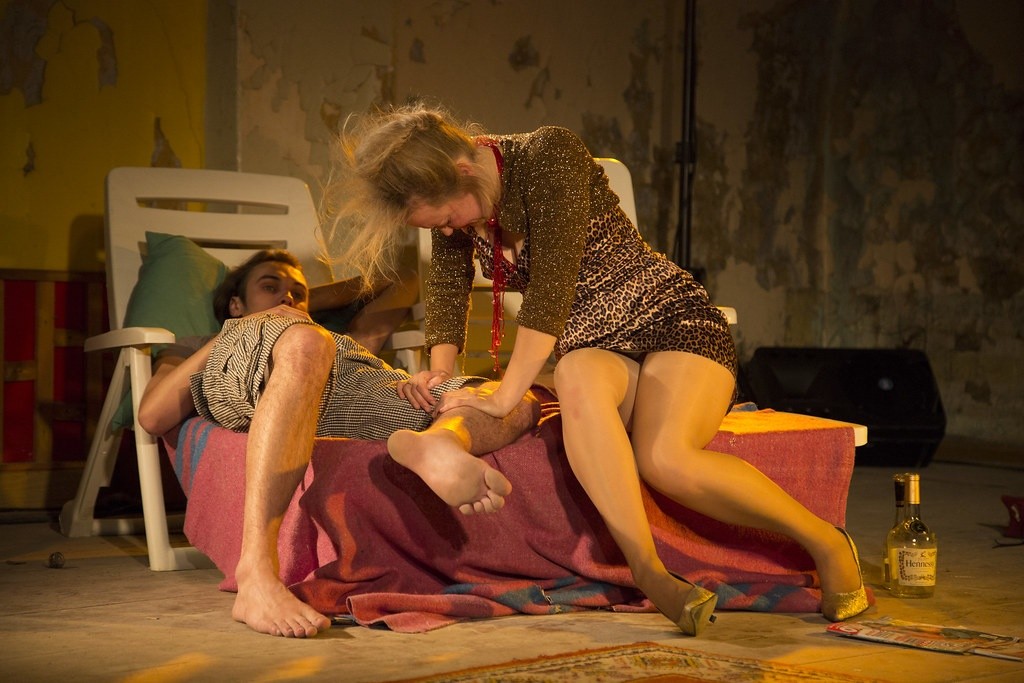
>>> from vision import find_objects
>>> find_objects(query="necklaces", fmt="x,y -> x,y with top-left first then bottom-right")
461,134 -> 514,373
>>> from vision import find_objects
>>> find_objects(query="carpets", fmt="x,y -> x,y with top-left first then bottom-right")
377,642 -> 892,683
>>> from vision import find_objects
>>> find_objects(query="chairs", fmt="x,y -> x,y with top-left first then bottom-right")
55,165 -> 341,570
401,155 -> 739,385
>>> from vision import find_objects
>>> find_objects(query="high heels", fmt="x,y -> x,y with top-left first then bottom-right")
813,526 -> 871,620
650,568 -> 719,635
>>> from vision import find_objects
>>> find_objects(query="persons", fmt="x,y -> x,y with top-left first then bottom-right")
317,104 -> 868,635
138,248 -> 542,639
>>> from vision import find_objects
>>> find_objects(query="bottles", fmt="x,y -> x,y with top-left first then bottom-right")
887,473 -> 936,598
882,475 -> 907,588
